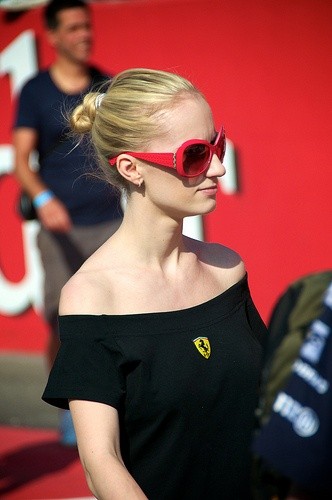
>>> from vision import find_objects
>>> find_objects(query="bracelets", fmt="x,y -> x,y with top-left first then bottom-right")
32,187 -> 54,209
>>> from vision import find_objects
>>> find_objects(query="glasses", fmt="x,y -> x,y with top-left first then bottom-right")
110,123 -> 229,176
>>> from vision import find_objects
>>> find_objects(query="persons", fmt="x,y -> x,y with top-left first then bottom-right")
14,0 -> 123,448
41,68 -> 269,500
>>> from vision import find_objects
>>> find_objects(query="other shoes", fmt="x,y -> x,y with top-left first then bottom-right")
59,409 -> 77,447
